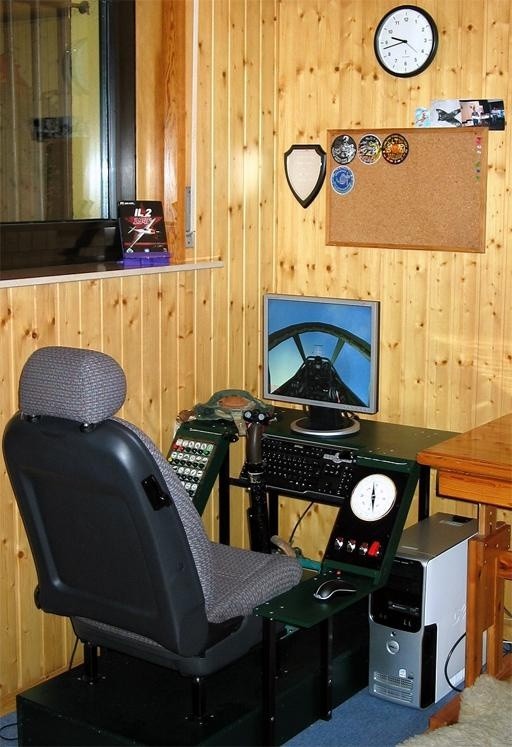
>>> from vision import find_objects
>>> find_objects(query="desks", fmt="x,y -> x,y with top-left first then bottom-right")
416,411 -> 512,733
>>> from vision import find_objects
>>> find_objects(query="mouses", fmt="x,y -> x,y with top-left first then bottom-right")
313,578 -> 359,601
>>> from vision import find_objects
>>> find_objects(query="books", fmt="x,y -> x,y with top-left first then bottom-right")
118,199 -> 170,260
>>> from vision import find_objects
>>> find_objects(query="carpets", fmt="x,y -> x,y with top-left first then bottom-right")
393,672 -> 511,747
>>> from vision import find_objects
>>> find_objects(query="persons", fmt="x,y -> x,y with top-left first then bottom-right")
491,106 -> 499,127
472,107 -> 480,126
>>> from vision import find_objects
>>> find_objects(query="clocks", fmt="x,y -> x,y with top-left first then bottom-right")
373,4 -> 438,78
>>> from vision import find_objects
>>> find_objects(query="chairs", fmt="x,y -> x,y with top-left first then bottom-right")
0,346 -> 303,747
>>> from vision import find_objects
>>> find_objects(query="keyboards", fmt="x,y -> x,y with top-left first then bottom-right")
237,437 -> 360,508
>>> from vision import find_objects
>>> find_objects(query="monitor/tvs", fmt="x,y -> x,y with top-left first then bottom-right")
262,293 -> 380,437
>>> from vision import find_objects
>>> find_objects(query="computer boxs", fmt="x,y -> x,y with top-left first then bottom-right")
369,511 -> 486,711
